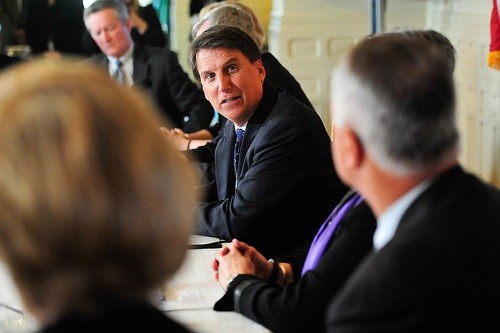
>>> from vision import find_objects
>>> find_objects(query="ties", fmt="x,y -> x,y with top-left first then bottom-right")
233,128 -> 246,174
110,60 -> 125,87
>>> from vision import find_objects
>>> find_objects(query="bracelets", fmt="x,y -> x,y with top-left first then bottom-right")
187,139 -> 192,151
267,258 -> 278,286
279,264 -> 287,286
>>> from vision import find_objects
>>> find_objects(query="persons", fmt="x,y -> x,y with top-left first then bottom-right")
213,26 -> 456,333
84,0 -> 215,134
159,5 -> 313,150
0,61 -> 197,333
0,0 -> 85,68
325,33 -> 500,333
179,24 -> 351,262
